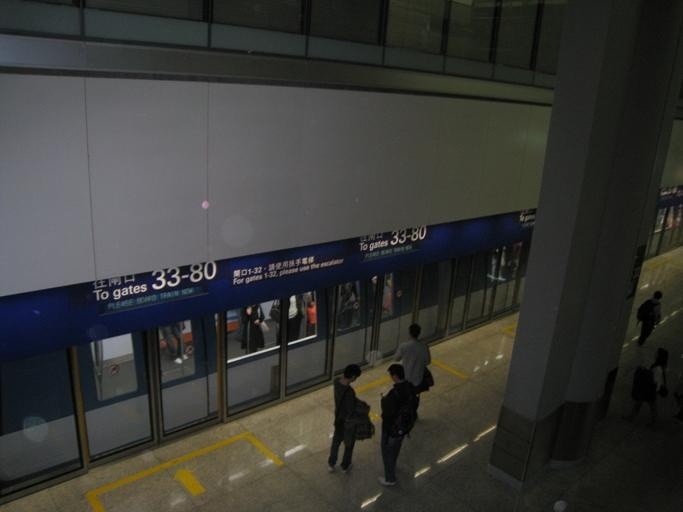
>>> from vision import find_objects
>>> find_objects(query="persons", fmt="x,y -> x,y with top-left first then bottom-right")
327,365 -> 362,472
636,290 -> 664,347
378,363 -> 416,487
624,347 -> 668,425
392,321 -> 429,421
160,275 -> 390,364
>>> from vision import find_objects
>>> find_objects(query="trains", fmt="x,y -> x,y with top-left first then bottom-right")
1,187 -> 683,501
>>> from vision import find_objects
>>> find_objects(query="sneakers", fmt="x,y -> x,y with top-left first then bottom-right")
377,476 -> 395,486
173,354 -> 188,363
328,463 -> 352,474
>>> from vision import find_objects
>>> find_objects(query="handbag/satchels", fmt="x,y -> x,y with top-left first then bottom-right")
271,305 -> 280,319
660,385 -> 669,398
393,404 -> 417,437
423,368 -> 434,391
260,321 -> 269,333
348,402 -> 374,439
632,366 -> 655,400
637,299 -> 657,324
